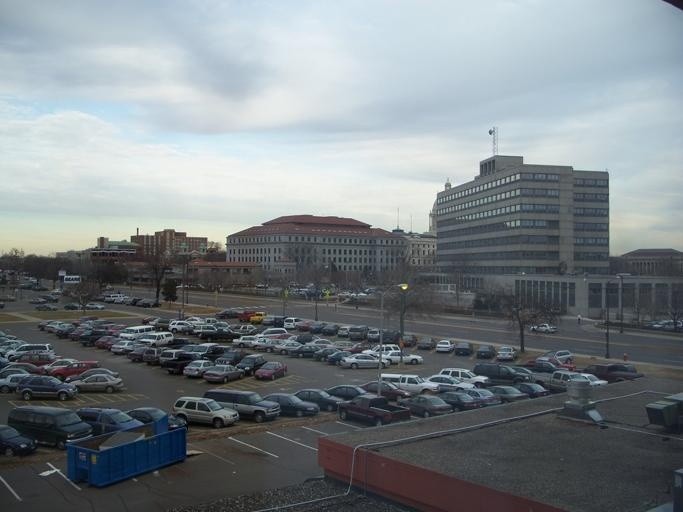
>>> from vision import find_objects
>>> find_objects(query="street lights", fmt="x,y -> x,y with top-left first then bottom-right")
186,259 -> 201,303
372,282 -> 411,399
614,273 -> 624,335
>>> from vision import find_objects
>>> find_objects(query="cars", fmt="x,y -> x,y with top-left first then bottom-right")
247,280 -> 392,306
0,269 -> 165,317
646,317 -> 681,330
